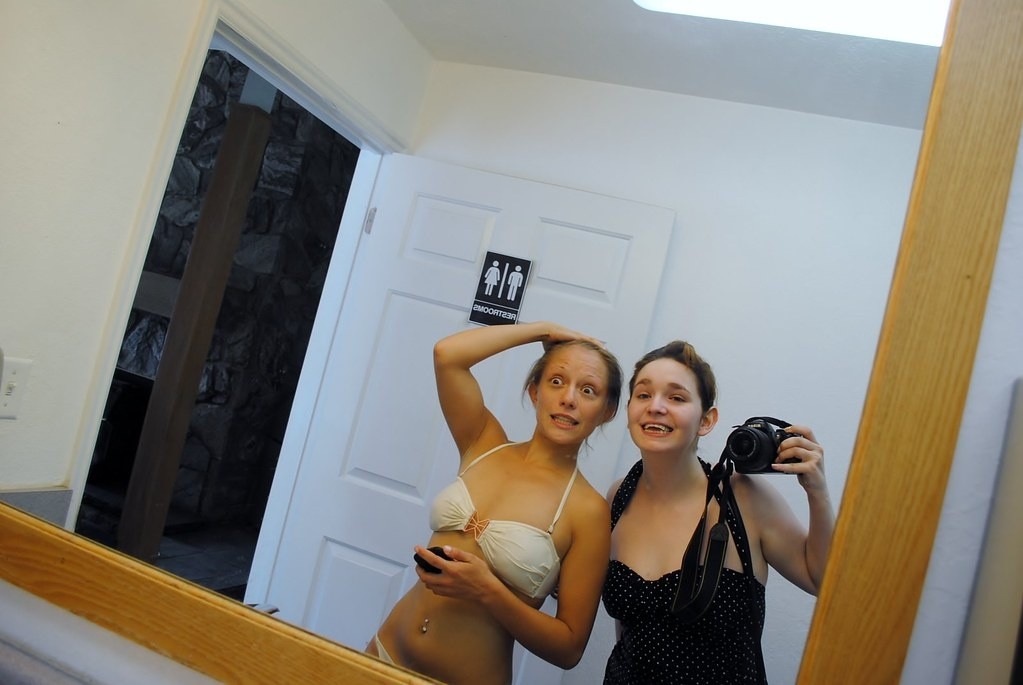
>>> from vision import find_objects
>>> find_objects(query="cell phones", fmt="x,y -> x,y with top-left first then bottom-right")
413,547 -> 455,574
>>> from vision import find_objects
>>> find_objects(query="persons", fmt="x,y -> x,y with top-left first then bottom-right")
365,320 -> 624,685
602,340 -> 835,685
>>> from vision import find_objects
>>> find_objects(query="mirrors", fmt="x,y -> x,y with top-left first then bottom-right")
0,0 -> 1023,685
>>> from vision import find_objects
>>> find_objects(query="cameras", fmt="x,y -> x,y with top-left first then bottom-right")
725,416 -> 804,474
